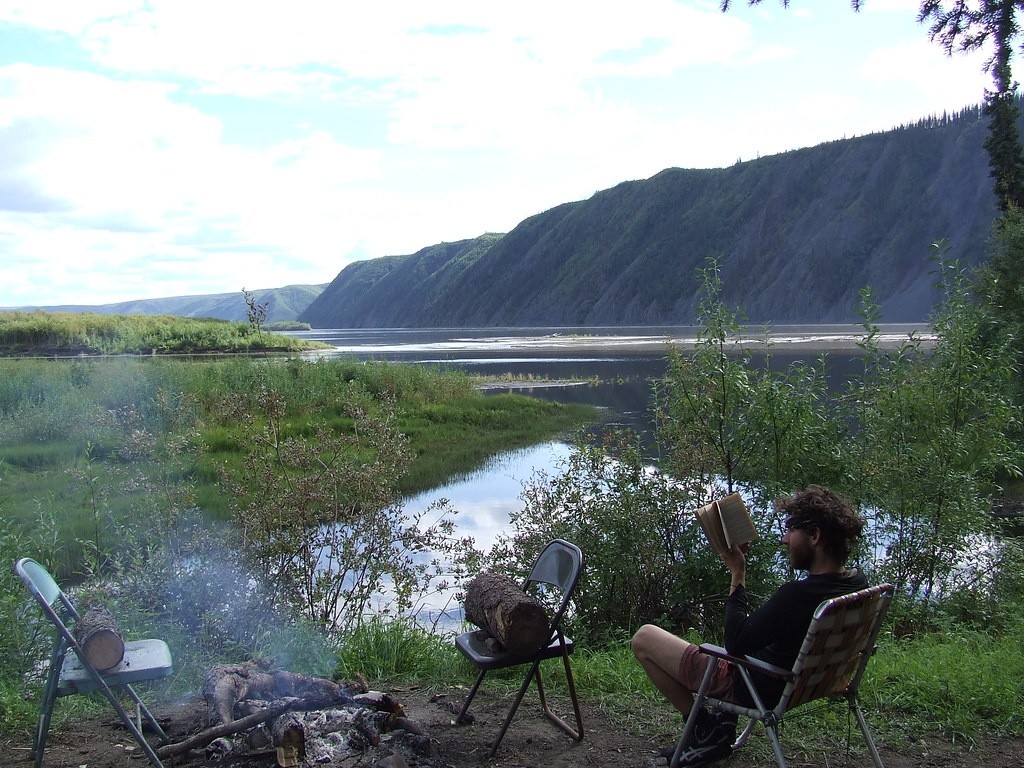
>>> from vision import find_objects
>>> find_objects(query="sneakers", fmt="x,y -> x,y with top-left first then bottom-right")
710,706 -> 739,735
661,720 -> 732,768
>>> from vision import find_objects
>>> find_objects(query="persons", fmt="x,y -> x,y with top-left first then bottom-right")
630,485 -> 869,768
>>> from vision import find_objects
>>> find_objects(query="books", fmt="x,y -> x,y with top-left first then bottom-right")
694,492 -> 758,555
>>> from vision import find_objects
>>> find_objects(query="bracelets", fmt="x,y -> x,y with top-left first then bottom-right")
731,584 -> 737,588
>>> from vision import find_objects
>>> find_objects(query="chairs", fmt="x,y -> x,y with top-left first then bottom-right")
455,539 -> 585,758
664,581 -> 894,767
14,556 -> 171,768
